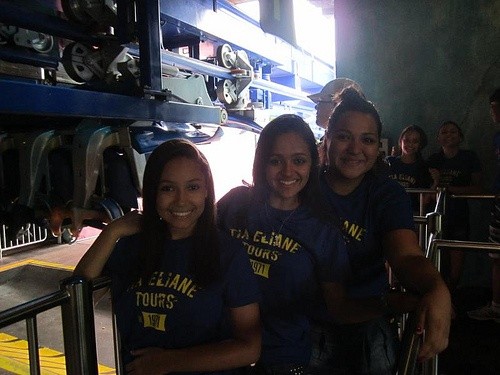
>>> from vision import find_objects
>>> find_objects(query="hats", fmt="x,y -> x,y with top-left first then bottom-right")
307,78 -> 365,102
489,87 -> 500,102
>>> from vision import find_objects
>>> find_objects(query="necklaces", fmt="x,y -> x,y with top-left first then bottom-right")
265,189 -> 296,238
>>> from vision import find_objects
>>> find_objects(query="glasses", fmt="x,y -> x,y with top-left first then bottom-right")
488,104 -> 500,110
314,96 -> 332,104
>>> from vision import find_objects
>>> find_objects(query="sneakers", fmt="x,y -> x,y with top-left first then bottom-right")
466,301 -> 500,322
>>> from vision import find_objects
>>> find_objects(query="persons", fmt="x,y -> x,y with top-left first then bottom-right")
215,115 -> 456,375
73,139 -> 262,375
25,121 -> 138,238
308,87 -> 451,375
314,79 -> 500,322
